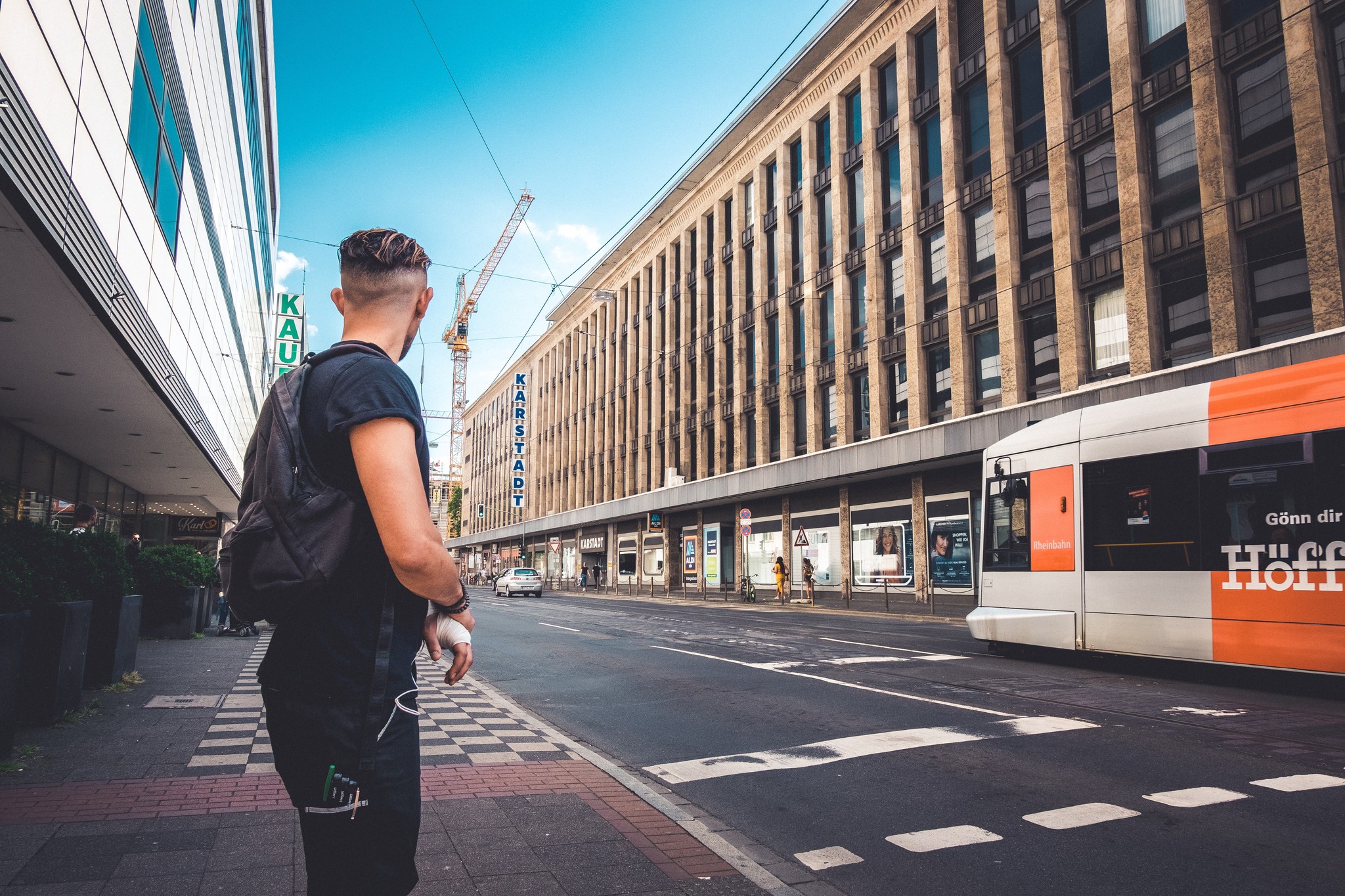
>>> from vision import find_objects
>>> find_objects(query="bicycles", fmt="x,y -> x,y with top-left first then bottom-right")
469,574 -> 479,585
738,574 -> 759,603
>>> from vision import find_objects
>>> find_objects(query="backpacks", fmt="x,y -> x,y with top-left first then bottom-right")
214,343 -> 396,774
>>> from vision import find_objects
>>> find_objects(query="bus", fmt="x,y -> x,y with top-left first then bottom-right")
966,350 -> 1345,686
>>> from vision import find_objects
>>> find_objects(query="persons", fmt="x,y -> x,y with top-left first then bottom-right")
930,521 -> 953,563
592,561 -> 602,590
69,503 -> 98,536
822,533 -> 827,543
196,550 -> 202,555
771,556 -> 786,600
481,568 -> 487,584
579,562 -> 591,591
1137,499 -> 1150,518
870,527 -> 903,583
802,558 -> 815,599
218,598 -> 229,626
124,533 -> 141,566
254,228 -> 476,896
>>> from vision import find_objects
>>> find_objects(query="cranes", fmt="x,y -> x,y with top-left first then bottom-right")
441,179 -> 535,540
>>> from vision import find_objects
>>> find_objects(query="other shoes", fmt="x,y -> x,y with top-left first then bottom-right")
582,589 -> 585,591
583,588 -> 585,590
805,598 -> 810,599
593,588 -> 597,590
218,624 -> 225,629
596,588 -> 598,590
230,623 -> 241,632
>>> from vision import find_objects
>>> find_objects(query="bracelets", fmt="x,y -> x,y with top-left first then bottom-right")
432,576 -> 470,614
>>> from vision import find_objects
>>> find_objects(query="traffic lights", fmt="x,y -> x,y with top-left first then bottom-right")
519,548 -> 526,559
478,504 -> 485,518
518,536 -> 523,548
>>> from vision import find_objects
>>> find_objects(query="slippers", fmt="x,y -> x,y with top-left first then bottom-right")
774,597 -> 779,599
780,598 -> 786,600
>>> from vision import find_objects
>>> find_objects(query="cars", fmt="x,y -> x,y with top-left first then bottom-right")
495,567 -> 542,598
492,568 -> 510,591
